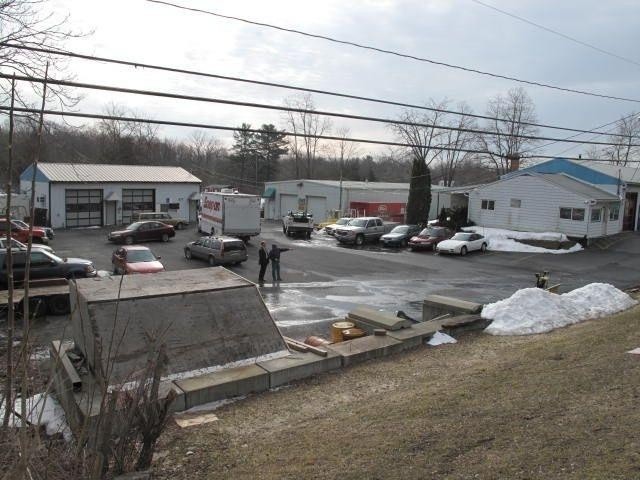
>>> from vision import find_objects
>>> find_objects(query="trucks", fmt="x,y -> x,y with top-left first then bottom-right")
197,192 -> 261,244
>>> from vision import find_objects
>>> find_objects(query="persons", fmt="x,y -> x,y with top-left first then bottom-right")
267,243 -> 296,282
257,240 -> 270,283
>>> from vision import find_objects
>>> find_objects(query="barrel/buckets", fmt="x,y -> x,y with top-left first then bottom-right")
304,322 -> 366,347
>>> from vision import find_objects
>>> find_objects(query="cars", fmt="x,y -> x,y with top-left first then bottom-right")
112,245 -> 166,275
0,218 -> 97,289
108,213 -> 190,245
325,217 -> 488,256
282,211 -> 313,238
184,236 -> 248,265
